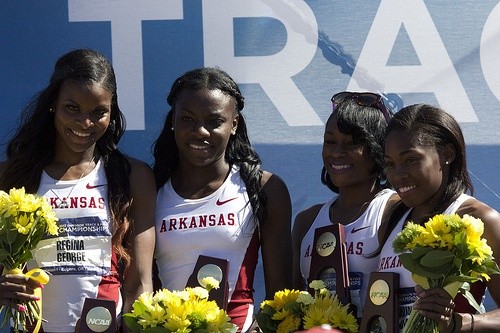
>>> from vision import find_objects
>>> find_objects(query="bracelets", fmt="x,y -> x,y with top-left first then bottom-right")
469,312 -> 476,333
451,314 -> 457,333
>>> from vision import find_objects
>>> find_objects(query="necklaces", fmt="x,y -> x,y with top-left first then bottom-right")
329,194 -> 367,226
50,152 -> 97,207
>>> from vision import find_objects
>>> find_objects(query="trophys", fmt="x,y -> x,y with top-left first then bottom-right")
77,298 -> 118,333
358,269 -> 400,333
183,255 -> 230,313
307,224 -> 357,315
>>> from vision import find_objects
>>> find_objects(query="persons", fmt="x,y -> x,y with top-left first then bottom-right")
284,89 -> 500,333
149,68 -> 293,333
0,49 -> 157,333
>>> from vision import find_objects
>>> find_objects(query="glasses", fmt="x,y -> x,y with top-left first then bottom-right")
331,92 -> 391,125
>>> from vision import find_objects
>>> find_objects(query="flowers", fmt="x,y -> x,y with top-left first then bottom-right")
255,279 -> 360,333
120,277 -> 240,333
0,185 -> 59,333
393,212 -> 500,333
